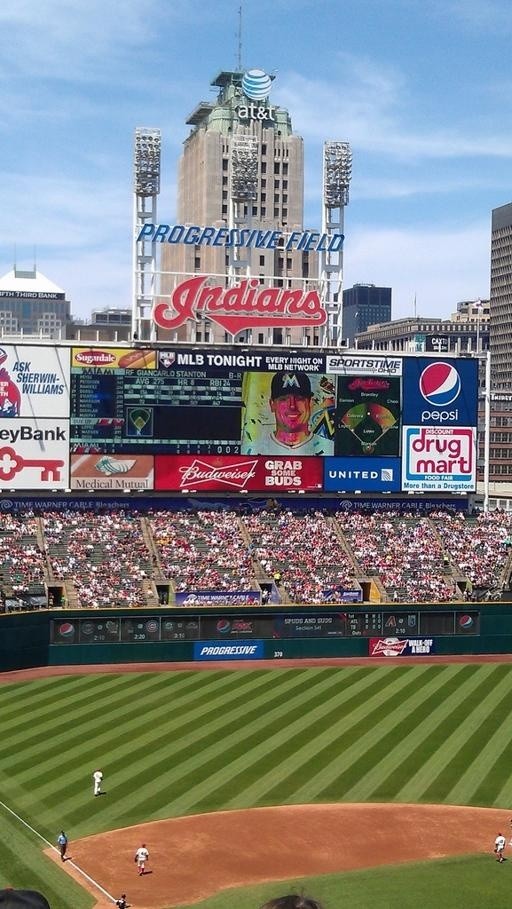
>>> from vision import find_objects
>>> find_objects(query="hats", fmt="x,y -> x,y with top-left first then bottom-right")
269,371 -> 315,398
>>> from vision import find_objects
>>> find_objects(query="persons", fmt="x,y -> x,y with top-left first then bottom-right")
0,888 -> 50,909
134,844 -> 150,876
259,895 -> 320,909
494,833 -> 507,863
247,371 -> 335,456
115,894 -> 134,909
1,503 -> 512,613
92,768 -> 103,796
58,831 -> 68,863
